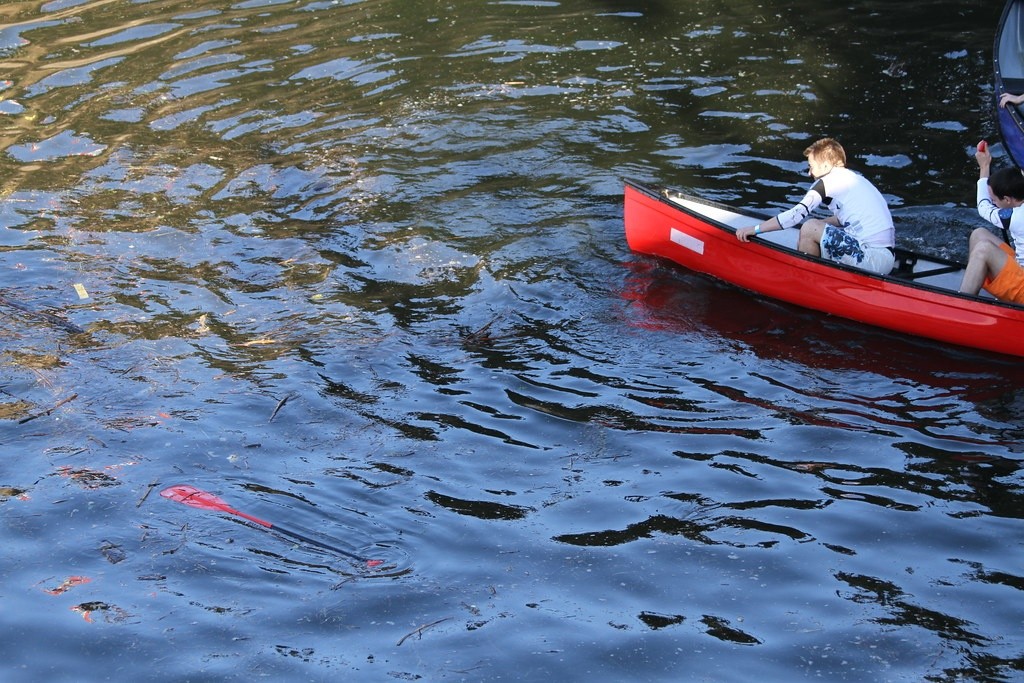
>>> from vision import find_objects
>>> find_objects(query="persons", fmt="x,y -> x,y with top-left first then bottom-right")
999,93 -> 1024,109
735,138 -> 896,274
959,142 -> 1024,305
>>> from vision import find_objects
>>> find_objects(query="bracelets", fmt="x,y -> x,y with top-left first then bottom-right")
755,225 -> 761,234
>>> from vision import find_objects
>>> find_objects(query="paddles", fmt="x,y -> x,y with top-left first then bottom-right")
161,483 -> 383,569
977,138 -> 1011,246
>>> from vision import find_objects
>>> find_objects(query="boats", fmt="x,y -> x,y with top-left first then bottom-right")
620,179 -> 1023,370
